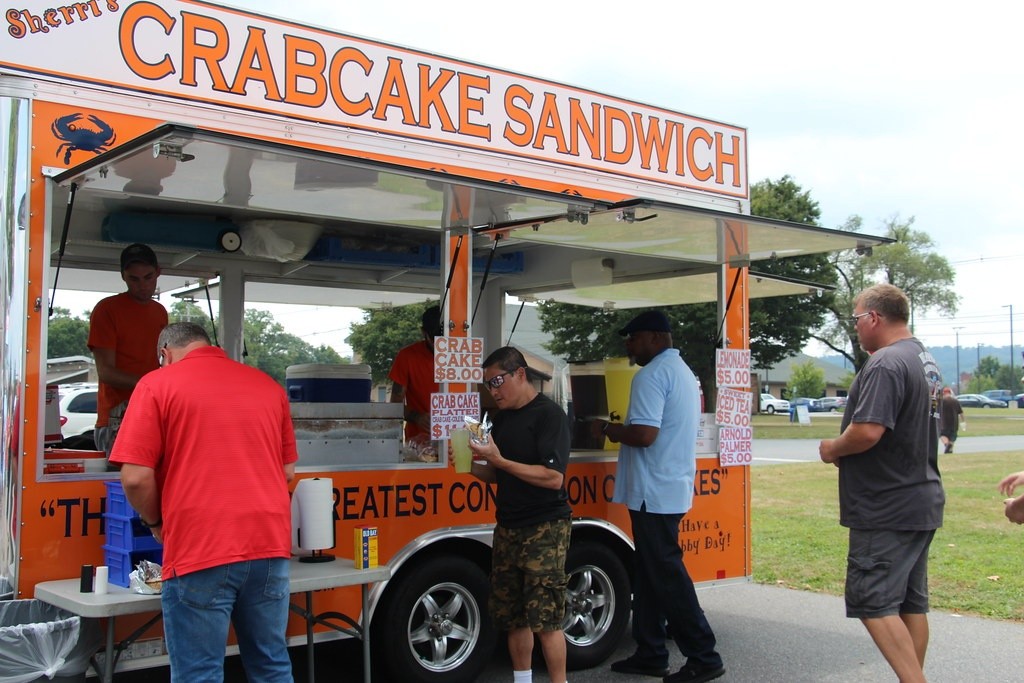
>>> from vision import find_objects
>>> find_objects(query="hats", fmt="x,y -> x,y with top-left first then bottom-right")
943,388 -> 951,393
421,305 -> 441,340
618,311 -> 670,337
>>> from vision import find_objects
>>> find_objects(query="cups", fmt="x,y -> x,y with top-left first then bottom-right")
449,428 -> 473,473
377,385 -> 386,402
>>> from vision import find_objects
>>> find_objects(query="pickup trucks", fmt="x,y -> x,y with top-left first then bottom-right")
982,390 -> 1024,408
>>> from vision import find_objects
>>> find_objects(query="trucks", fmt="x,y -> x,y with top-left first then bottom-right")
0,0 -> 900,683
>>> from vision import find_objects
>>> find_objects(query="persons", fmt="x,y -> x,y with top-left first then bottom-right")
109,321 -> 299,683
448,346 -> 573,683
387,307 -> 443,447
819,285 -> 947,683
998,471 -> 1024,525
589,309 -> 726,683
939,388 -> 964,454
85,243 -> 169,452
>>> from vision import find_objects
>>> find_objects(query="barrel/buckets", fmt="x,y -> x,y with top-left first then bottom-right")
286,362 -> 374,402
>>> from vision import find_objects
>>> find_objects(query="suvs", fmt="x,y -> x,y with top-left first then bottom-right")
53,383 -> 99,441
761,393 -> 790,414
791,395 -> 846,412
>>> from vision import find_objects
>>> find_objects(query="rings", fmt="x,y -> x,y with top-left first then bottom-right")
152,534 -> 156,537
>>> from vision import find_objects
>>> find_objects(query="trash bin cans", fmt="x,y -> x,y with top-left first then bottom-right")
0,576 -> 14,600
0,599 -> 86,683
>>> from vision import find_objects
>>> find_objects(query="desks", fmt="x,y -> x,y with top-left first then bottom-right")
35,553 -> 393,683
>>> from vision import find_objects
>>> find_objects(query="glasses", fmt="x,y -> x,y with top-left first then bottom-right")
483,369 -> 516,391
851,312 -> 883,325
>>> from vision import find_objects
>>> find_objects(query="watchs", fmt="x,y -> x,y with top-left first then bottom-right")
140,518 -> 162,528
601,422 -> 610,434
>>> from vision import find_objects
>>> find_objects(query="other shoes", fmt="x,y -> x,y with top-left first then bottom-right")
609,647 -> 670,677
943,443 -> 953,454
663,651 -> 726,683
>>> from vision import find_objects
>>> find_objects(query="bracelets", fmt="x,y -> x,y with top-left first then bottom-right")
407,411 -> 418,421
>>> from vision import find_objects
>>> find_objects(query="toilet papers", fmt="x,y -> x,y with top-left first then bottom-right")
290,477 -> 334,555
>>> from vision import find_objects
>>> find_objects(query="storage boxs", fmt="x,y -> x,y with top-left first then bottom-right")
101,547 -> 163,589
353,524 -> 380,570
291,400 -> 408,466
43,384 -> 63,445
285,361 -> 373,402
100,515 -> 163,549
44,446 -> 108,473
103,480 -> 145,519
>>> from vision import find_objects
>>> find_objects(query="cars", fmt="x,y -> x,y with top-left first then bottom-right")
957,393 -> 1007,408
